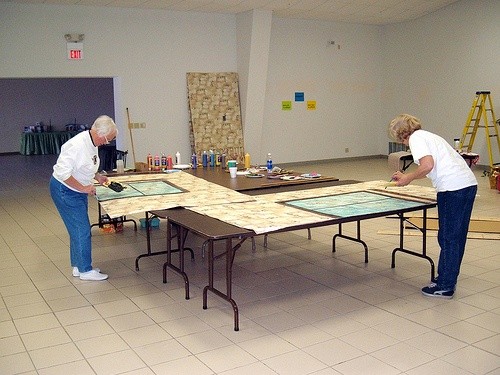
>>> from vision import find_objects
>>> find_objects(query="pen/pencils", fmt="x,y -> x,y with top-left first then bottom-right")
89,182 -> 96,195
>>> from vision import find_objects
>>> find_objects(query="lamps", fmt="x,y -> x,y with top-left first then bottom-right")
64,33 -> 86,60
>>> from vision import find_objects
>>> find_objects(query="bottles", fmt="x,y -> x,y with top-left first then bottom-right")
154,153 -> 160,171
266,152 -> 273,174
202,151 -> 207,167
175,152 -> 181,164
161,153 -> 167,170
245,153 -> 251,168
210,151 -> 214,167
147,154 -> 153,170
215,152 -> 221,165
191,152 -> 197,169
167,154 -> 173,170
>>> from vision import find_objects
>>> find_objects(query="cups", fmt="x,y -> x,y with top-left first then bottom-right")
229,167 -> 237,178
116,160 -> 124,174
228,160 -> 236,167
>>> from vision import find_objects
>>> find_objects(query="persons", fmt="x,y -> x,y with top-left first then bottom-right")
389,114 -> 478,299
49,115 -> 118,281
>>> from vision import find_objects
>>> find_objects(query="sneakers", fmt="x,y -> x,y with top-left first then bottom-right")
421,283 -> 455,299
431,275 -> 456,290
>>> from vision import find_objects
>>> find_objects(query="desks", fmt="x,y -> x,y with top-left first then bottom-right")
388,151 -> 479,172
91,164 -> 439,331
20,131 -> 83,154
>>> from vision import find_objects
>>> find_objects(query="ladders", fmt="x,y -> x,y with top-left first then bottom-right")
458,91 -> 500,171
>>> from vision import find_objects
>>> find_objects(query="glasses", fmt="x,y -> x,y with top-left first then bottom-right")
104,136 -> 110,145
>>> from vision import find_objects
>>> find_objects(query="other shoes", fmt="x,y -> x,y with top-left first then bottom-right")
79,270 -> 108,280
72,267 -> 100,276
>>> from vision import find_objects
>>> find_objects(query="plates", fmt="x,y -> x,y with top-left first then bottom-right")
300,173 -> 321,178
247,174 -> 264,178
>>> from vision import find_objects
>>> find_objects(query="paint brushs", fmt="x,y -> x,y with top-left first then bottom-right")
385,172 -> 399,189
